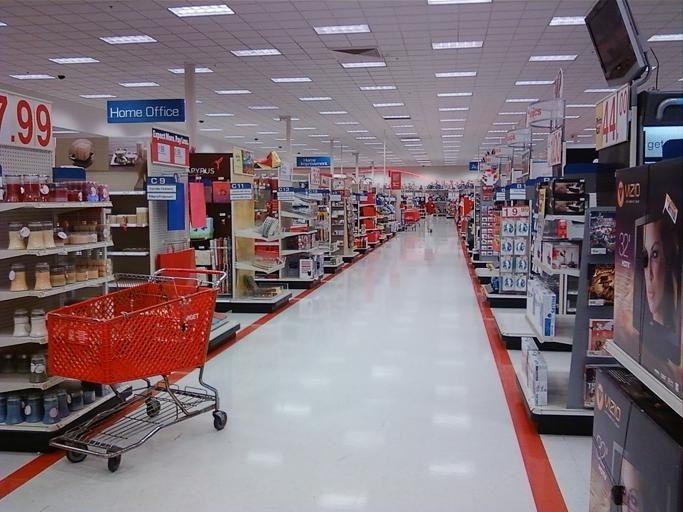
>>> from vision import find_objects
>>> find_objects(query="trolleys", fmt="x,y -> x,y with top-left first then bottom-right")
47,268 -> 227,473
403,207 -> 420,231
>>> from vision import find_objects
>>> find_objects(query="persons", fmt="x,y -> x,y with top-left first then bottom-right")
423,196 -> 440,234
616,458 -> 665,511
639,221 -> 677,347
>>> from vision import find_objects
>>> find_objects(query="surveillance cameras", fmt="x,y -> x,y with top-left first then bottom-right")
57,73 -> 66,80
199,120 -> 301,155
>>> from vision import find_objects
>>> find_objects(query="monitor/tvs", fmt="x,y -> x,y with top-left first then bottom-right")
583,0 -> 650,85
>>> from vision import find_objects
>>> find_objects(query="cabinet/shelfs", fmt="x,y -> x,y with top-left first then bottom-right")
0,156 -> 360,456
455,84 -> 682,507
359,172 -> 453,252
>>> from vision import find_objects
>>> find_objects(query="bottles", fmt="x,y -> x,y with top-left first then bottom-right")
1,174 -> 110,425
